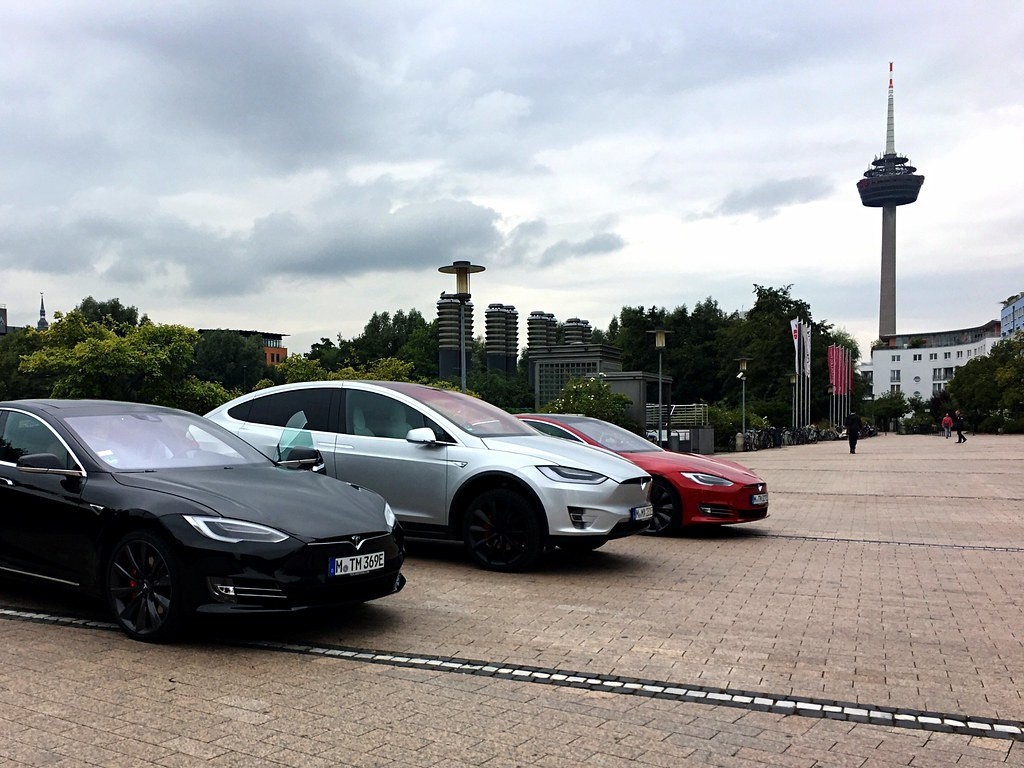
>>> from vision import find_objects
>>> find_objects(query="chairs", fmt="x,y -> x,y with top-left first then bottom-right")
388,404 -> 412,438
351,408 -> 376,438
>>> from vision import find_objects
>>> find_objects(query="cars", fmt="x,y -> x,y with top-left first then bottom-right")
0,400 -> 408,643
183,380 -> 657,571
464,413 -> 769,537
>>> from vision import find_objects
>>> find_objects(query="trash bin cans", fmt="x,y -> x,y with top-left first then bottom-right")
736,432 -> 745,451
668,430 -> 680,451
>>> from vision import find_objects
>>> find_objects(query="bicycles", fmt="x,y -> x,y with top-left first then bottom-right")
729,425 -> 848,452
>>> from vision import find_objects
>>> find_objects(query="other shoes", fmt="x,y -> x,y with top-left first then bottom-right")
946,435 -> 949,439
955,441 -> 961,443
962,439 -> 967,443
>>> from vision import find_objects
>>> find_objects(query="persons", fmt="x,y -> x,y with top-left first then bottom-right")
845,407 -> 863,453
942,412 -> 953,439
954,410 -> 967,443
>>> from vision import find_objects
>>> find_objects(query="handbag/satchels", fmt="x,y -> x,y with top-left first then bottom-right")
944,423 -> 949,428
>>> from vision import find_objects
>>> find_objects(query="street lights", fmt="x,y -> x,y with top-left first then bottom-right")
784,373 -> 802,430
437,260 -> 486,391
825,384 -> 835,429
732,357 -> 755,436
646,329 -> 678,453
